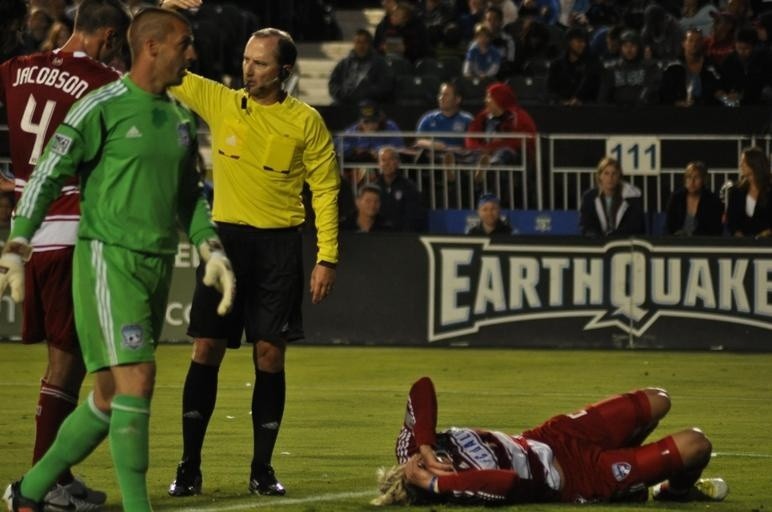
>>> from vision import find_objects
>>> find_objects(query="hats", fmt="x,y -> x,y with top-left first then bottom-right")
478,193 -> 500,208
488,84 -> 516,109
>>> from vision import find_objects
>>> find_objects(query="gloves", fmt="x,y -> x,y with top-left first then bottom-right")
0,253 -> 26,305
200,238 -> 237,316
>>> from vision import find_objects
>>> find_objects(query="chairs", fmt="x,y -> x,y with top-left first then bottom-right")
390,0 -> 693,109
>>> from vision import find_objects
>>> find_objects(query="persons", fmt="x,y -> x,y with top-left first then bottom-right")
0,6 -> 236,512
327,1 -> 771,241
369,376 -> 729,507
1,0 -> 342,236
0,1 -> 128,512
160,1 -> 340,498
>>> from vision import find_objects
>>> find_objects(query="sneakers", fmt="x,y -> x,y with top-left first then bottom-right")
168,462 -> 202,496
653,479 -> 727,501
2,476 -> 106,512
249,467 -> 285,496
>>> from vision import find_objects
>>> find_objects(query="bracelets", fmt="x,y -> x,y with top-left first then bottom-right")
429,476 -> 437,494
